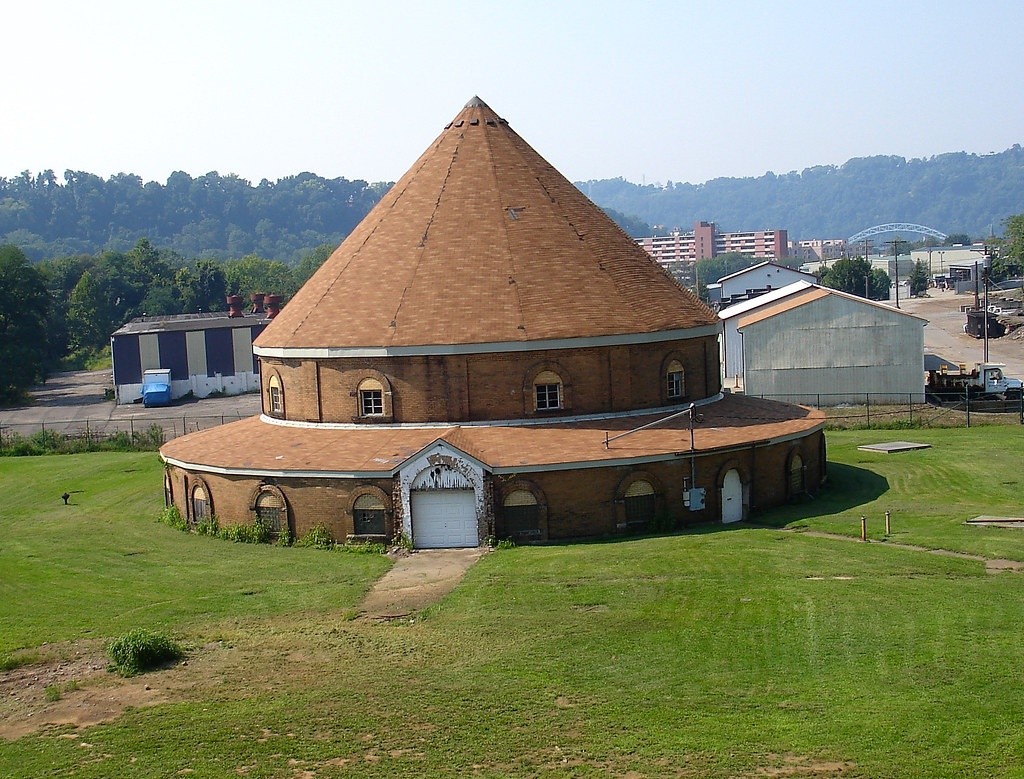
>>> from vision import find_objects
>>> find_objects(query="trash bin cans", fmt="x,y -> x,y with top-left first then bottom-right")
965,310 -> 999,339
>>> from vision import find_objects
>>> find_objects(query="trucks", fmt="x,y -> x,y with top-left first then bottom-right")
953,364 -> 1024,401
141,369 -> 172,407
